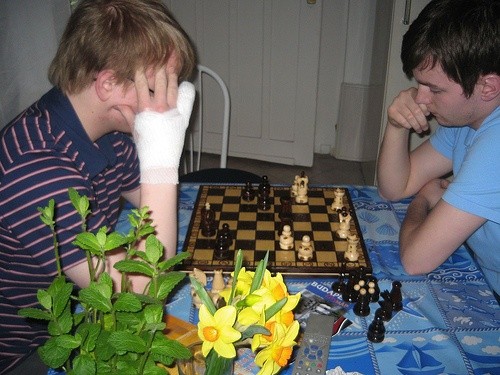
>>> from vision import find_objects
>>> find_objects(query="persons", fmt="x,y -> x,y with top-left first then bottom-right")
0,0 -> 196,375
376,0 -> 500,303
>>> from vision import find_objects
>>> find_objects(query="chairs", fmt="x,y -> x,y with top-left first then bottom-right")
182,65 -> 231,176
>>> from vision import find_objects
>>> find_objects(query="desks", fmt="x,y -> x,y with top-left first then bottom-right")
47,182 -> 500,375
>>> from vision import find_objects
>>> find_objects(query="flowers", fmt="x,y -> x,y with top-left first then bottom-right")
188,248 -> 302,375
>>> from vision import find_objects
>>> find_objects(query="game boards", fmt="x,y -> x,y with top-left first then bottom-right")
174,185 -> 373,278
